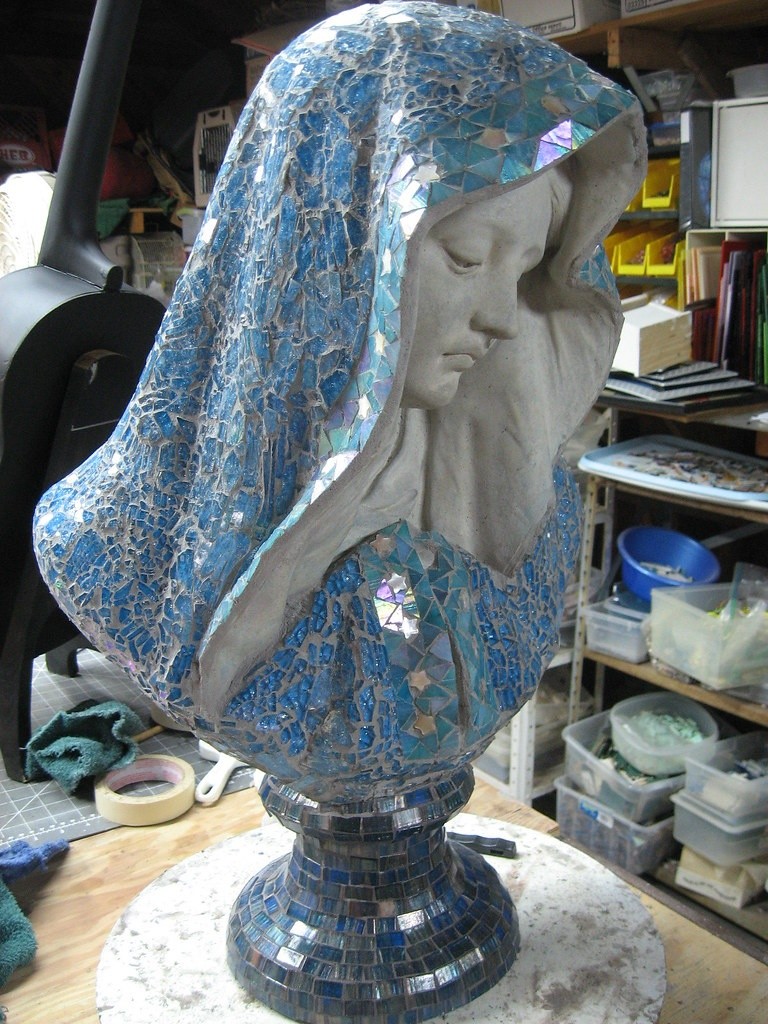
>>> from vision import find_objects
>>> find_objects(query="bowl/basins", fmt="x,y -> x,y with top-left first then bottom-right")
615,526 -> 721,601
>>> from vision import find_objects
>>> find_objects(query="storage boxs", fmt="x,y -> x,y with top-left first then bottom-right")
678,731 -> 768,822
561,711 -> 686,822
673,845 -> 768,909
553,773 -> 675,877
577,602 -> 650,664
647,586 -> 768,692
670,792 -> 768,870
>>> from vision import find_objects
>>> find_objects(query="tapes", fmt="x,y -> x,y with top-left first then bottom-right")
93,753 -> 197,827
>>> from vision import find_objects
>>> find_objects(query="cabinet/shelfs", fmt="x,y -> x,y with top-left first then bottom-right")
564,473 -> 768,940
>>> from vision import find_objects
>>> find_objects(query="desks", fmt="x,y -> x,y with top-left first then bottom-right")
0,649 -> 768,1024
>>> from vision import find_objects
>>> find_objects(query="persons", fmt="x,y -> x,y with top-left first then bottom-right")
31,2 -> 650,789
